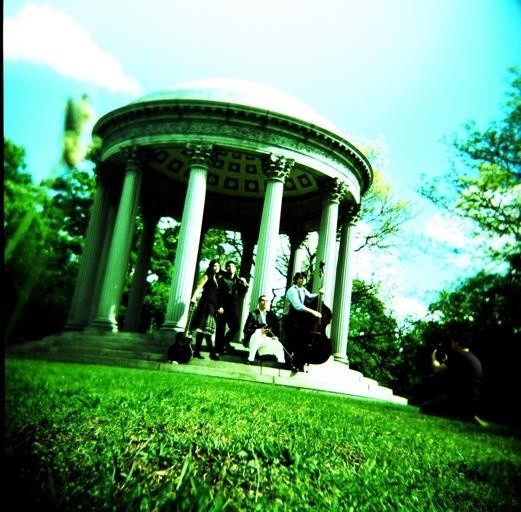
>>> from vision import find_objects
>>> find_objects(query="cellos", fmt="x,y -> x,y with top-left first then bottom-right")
300,260 -> 332,364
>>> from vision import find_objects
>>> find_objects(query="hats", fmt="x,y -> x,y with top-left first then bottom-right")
293,271 -> 308,282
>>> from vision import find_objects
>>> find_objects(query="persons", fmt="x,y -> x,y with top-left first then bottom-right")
283,271 -> 326,370
412,327 -> 491,428
188,258 -> 226,361
242,294 -> 289,369
211,259 -> 251,356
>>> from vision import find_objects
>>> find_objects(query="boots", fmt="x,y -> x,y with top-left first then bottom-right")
205,335 -> 221,360
193,333 -> 204,359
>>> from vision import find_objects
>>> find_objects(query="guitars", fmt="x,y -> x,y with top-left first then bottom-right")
168,302 -> 195,363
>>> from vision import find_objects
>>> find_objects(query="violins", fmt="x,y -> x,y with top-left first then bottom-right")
253,323 -> 268,330
